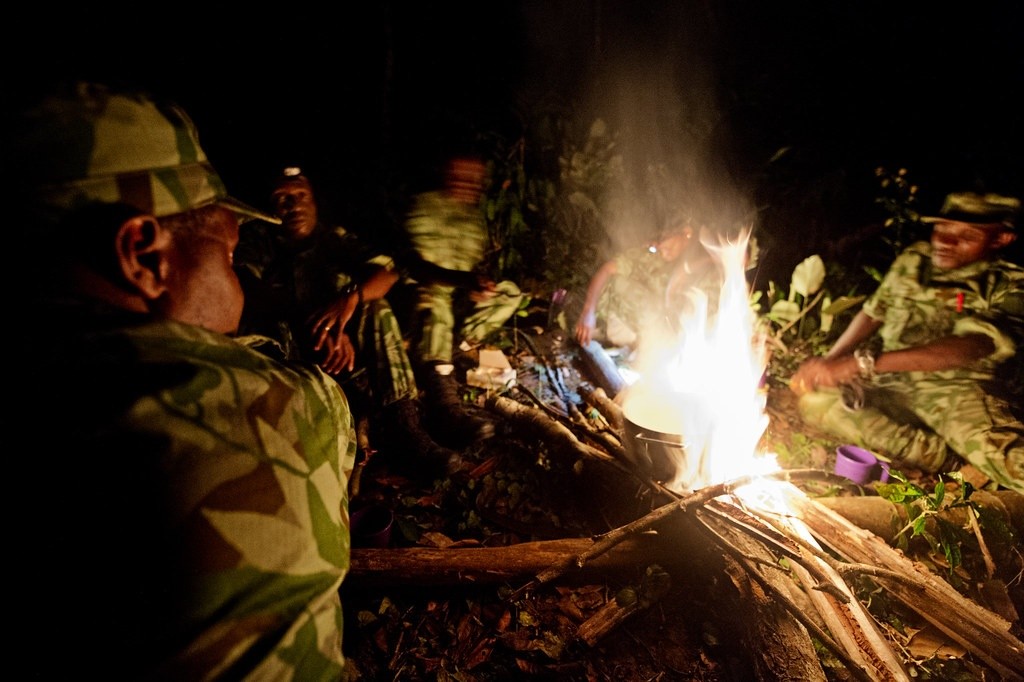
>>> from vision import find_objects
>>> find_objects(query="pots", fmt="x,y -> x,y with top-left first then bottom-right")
620,389 -> 688,481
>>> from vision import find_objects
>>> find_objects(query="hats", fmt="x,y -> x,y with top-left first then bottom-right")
15,81 -> 283,235
921,193 -> 1016,230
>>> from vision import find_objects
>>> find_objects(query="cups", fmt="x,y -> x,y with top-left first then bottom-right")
349,504 -> 393,547
836,445 -> 891,485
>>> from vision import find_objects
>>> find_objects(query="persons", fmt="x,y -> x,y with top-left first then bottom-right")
793,167 -> 1024,498
0,85 -> 528,682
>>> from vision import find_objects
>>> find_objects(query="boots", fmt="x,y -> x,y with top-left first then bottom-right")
351,415 -> 394,549
383,394 -> 461,475
422,360 -> 495,445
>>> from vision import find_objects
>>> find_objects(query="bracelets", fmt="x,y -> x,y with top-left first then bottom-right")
357,288 -> 364,302
855,343 -> 875,377
